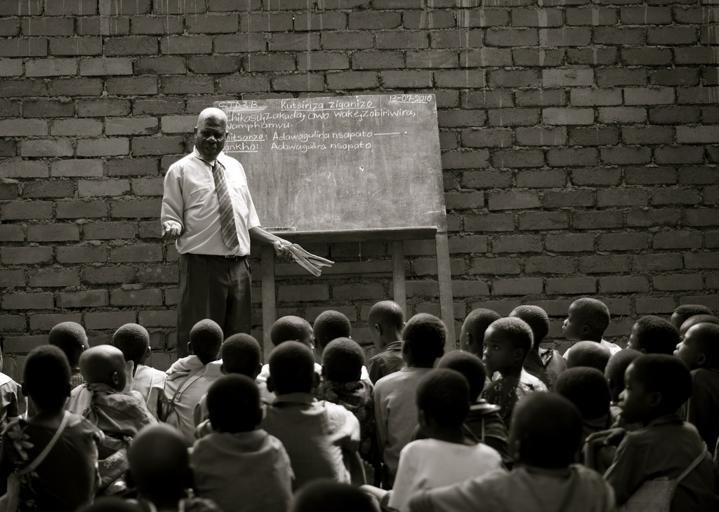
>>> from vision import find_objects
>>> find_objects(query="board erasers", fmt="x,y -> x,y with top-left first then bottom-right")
263,227 -> 297,233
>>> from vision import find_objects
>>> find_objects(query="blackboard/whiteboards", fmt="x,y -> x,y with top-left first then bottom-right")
212,92 -> 447,234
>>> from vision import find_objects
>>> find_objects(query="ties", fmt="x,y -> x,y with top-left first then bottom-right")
212,161 -> 240,257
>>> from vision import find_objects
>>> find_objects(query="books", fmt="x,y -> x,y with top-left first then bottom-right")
279,242 -> 336,278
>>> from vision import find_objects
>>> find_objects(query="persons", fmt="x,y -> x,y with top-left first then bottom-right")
159,107 -> 293,362
0,296 -> 719,512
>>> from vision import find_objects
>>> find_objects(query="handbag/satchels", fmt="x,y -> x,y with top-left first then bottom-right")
620,476 -> 675,512
